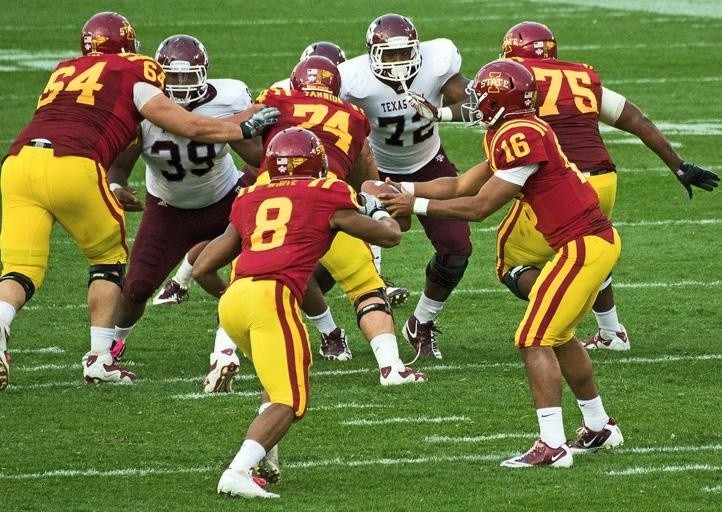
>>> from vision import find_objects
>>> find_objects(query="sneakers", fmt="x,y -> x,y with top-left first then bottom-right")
385,283 -> 408,305
569,417 -> 624,454
82,352 -> 136,386
217,467 -> 280,499
401,314 -> 443,365
579,324 -> 631,351
111,339 -> 126,361
379,357 -> 427,386
0,321 -> 9,391
319,326 -> 353,363
499,441 -> 574,470
153,279 -> 189,304
254,445 -> 281,484
203,349 -> 242,394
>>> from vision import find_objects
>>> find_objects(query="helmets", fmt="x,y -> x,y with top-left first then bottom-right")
265,128 -> 329,181
366,14 -> 422,81
461,61 -> 536,126
500,22 -> 556,61
299,42 -> 346,65
81,13 -> 140,55
155,34 -> 209,104
289,55 -> 342,98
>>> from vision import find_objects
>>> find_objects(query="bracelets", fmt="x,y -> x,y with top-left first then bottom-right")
414,197 -> 433,218
399,180 -> 416,195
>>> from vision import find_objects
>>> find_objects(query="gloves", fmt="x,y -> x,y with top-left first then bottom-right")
357,192 -> 394,220
407,90 -> 453,124
674,160 -> 720,199
241,106 -> 281,138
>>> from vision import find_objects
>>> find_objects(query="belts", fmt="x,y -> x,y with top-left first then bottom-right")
27,142 -> 52,148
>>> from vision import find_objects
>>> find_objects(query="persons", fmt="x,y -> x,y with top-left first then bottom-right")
376,60 -> 629,467
108,12 -> 476,395
187,122 -> 403,503
406,20 -> 722,351
0,11 -> 281,392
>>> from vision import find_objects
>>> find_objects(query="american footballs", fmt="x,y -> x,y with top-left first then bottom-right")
360,180 -> 411,232
